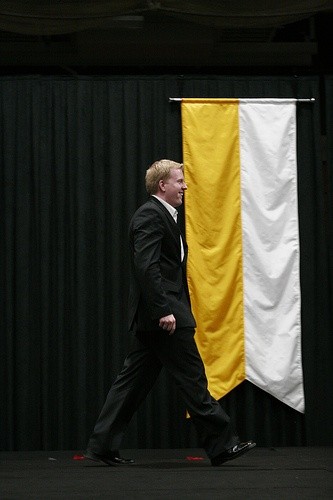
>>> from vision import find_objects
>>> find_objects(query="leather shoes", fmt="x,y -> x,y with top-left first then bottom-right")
85,448 -> 134,466
210,440 -> 256,467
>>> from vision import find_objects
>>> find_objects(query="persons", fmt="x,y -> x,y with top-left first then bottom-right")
83,159 -> 256,467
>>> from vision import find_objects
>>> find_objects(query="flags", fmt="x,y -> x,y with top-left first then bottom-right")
181,97 -> 305,418
